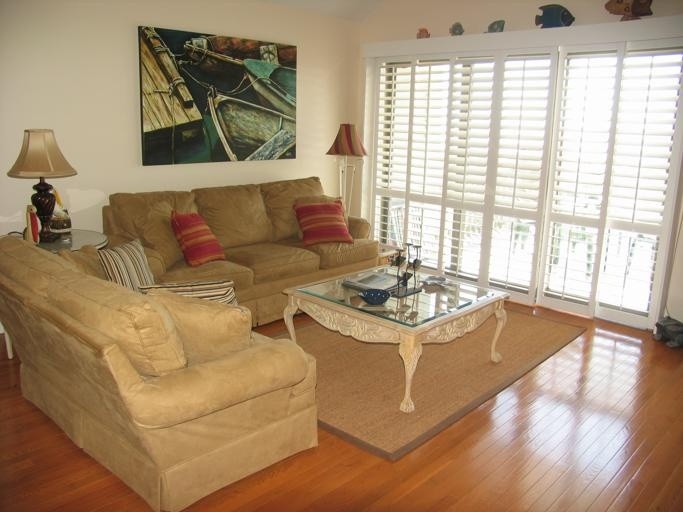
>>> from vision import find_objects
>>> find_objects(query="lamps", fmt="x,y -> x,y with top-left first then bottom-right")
7,128 -> 77,243
326,123 -> 366,214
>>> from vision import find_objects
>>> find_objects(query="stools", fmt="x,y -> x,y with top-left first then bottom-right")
5,228 -> 108,361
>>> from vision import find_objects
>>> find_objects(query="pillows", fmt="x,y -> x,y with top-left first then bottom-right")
139,280 -> 238,307
293,200 -> 353,245
96,238 -> 153,293
58,245 -> 105,279
174,210 -> 226,267
148,290 -> 252,366
135,213 -> 187,271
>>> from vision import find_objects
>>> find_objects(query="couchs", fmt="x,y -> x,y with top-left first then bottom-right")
0,232 -> 318,508
103,176 -> 379,328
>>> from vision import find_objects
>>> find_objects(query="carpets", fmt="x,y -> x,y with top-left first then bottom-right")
268,307 -> 586,462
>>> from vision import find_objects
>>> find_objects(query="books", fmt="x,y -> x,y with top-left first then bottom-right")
342,272 -> 407,294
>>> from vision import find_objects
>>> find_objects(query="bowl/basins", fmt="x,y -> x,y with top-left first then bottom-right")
358,289 -> 390,305
